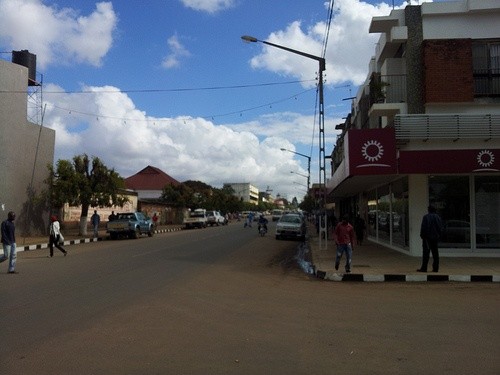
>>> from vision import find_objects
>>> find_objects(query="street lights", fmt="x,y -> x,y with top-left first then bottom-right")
290,171 -> 310,218
241,34 -> 329,251
279,147 -> 311,221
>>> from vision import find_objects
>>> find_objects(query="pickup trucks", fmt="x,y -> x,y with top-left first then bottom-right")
106,211 -> 155,240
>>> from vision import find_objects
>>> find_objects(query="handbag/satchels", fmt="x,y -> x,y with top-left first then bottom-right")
59,232 -> 65,242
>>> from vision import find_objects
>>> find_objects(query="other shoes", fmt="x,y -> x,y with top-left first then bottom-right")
8,271 -> 18,273
47,256 -> 53,258
336,268 -> 338,271
346,271 -> 351,273
64,251 -> 67,256
417,269 -> 426,272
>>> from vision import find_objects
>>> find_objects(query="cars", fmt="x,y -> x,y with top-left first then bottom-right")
206,210 -> 228,227
274,214 -> 308,242
239,208 -> 305,222
439,219 -> 492,245
368,210 -> 402,233
183,207 -> 209,228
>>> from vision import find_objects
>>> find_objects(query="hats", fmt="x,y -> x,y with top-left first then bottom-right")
51,216 -> 57,221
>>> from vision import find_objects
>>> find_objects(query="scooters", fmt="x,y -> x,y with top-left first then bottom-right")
257,221 -> 267,238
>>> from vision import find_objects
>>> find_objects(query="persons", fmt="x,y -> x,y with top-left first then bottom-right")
417,206 -> 443,272
301,213 -> 365,274
47,216 -> 67,258
0,211 -> 19,274
247,212 -> 269,233
91,209 -> 159,238
